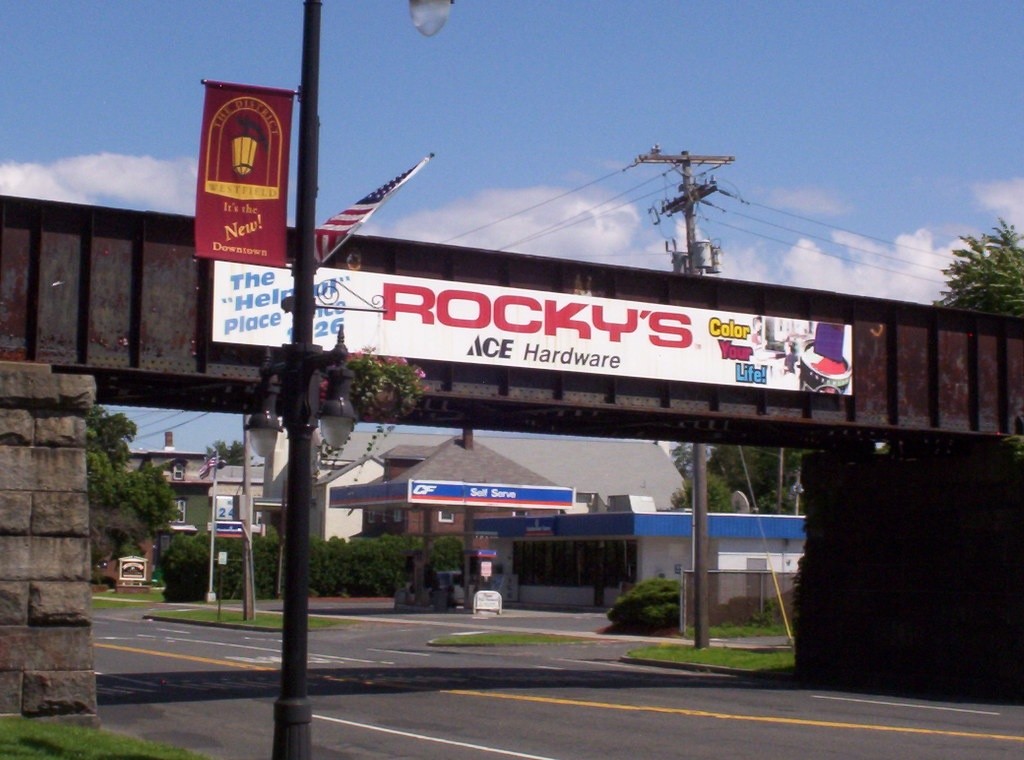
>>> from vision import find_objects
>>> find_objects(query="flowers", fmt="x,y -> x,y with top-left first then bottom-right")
319,346 -> 433,424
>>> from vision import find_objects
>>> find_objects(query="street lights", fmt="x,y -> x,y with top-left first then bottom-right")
204,448 -> 227,604
745,445 -> 787,515
247,0 -> 460,759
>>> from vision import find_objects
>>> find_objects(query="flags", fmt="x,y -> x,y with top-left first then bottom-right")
314,157 -> 429,263
199,451 -> 217,480
